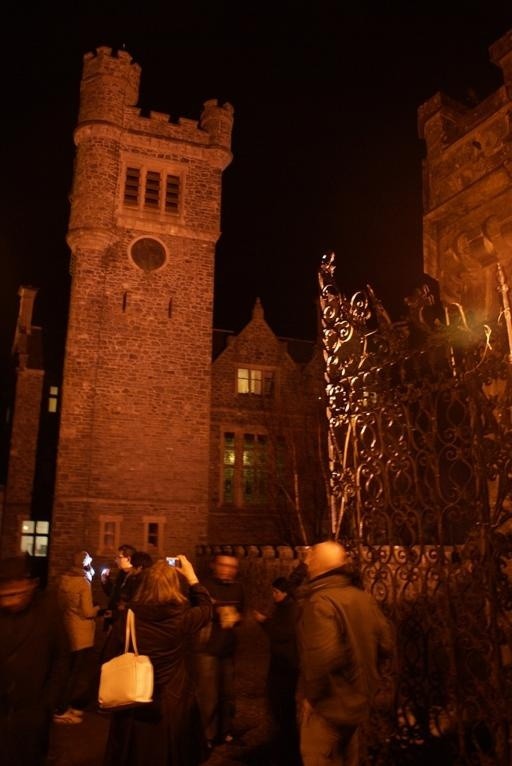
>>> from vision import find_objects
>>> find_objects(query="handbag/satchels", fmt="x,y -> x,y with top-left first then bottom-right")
98,653 -> 154,708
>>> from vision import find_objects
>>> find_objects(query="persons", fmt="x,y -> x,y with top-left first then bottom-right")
1,538 -> 405,765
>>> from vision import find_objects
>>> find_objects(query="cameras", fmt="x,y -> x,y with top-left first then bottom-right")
166,556 -> 180,568
99,567 -> 106,576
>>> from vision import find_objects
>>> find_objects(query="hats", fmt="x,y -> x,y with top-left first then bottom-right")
74,551 -> 92,568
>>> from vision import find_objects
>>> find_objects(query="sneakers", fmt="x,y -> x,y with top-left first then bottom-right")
220,733 -> 246,747
53,708 -> 83,725
205,741 -> 213,760
